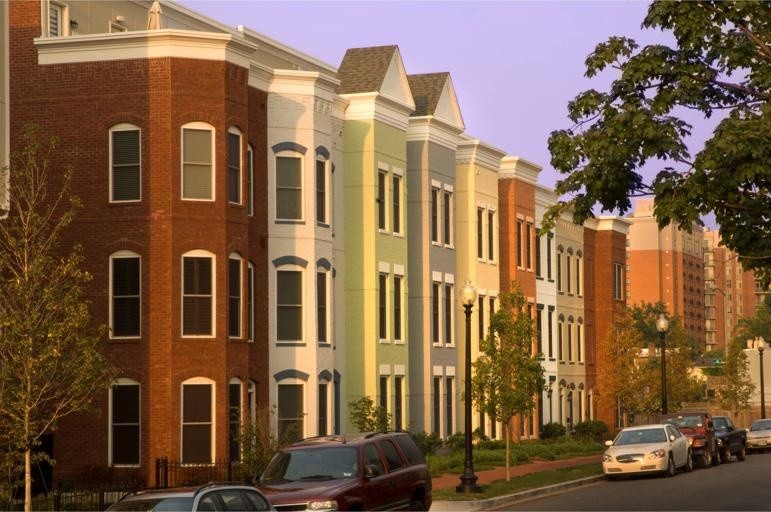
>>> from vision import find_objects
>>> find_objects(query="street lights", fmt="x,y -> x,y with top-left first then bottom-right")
656,314 -> 669,414
455,280 -> 482,493
758,339 -> 765,418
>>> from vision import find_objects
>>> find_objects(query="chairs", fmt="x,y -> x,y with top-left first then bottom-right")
298,462 -> 322,478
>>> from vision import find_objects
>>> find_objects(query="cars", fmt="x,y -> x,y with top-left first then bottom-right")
602,411 -> 771,481
